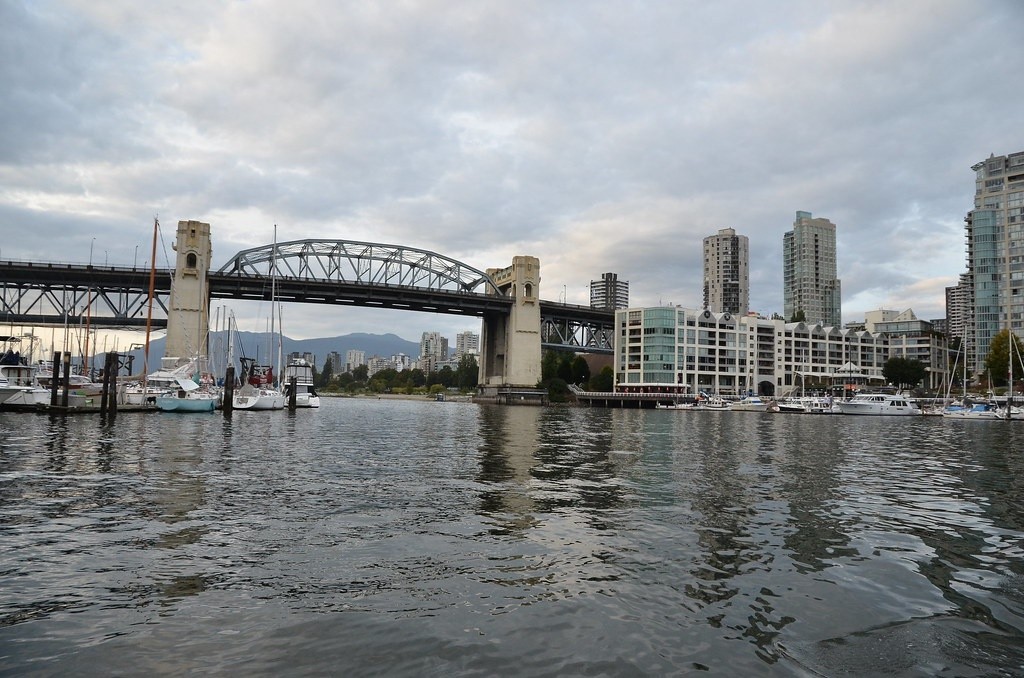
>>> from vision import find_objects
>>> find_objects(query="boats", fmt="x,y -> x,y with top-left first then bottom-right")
0,214 -> 321,411
835,393 -> 917,416
655,380 -> 834,414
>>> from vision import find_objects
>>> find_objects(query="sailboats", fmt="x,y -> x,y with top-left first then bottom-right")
920,320 -> 1024,419
